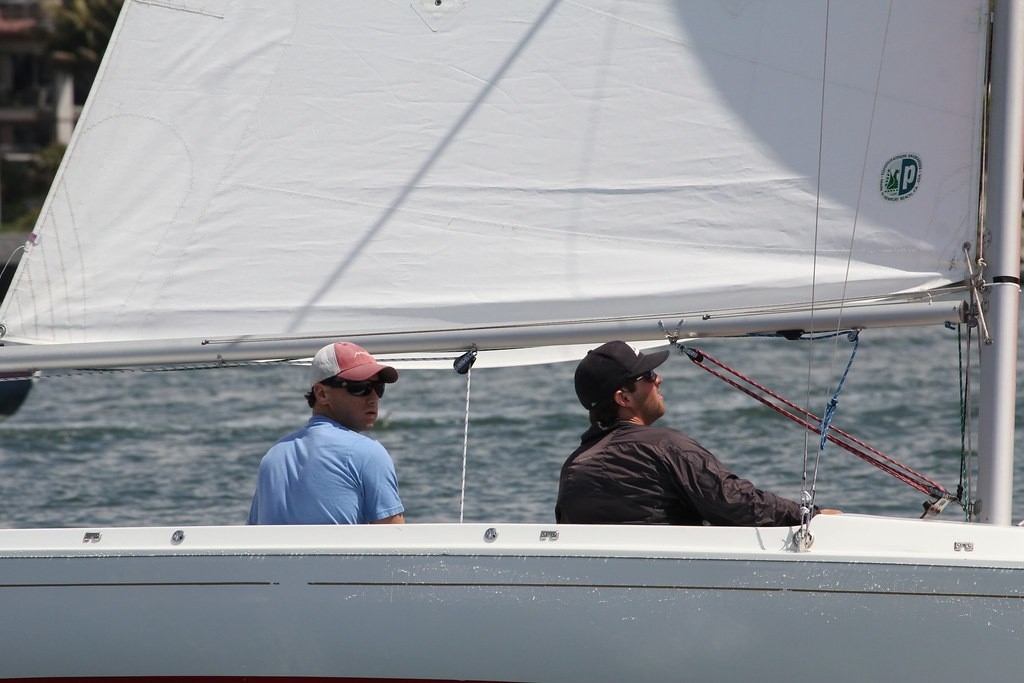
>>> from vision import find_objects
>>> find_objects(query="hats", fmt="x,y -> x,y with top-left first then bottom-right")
310,342 -> 398,388
574,340 -> 670,410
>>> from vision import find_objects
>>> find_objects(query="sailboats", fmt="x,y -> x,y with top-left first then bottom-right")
1,1 -> 1024,683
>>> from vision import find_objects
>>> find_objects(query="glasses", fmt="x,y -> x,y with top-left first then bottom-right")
610,370 -> 655,408
324,378 -> 385,398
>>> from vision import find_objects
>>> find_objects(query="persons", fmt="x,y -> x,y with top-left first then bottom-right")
554,341 -> 843,526
248,340 -> 408,525
